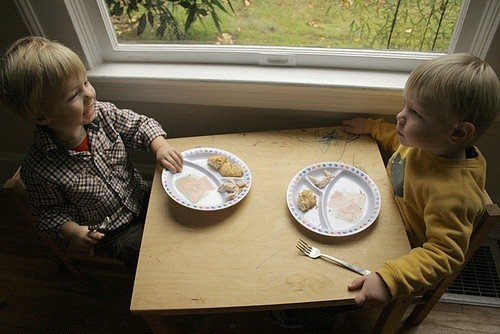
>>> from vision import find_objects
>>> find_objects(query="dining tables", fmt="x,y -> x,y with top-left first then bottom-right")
130,125 -> 424,334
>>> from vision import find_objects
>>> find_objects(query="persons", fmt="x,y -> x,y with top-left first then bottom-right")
269,52 -> 499,328
0,36 -> 196,334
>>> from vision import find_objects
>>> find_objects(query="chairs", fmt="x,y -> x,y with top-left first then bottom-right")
332,190 -> 500,334
2,166 -> 136,293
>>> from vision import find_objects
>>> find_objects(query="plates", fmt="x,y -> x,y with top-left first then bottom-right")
286,162 -> 381,236
161,147 -> 252,211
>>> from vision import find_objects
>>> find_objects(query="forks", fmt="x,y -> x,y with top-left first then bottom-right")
297,239 -> 371,277
88,214 -> 99,257
